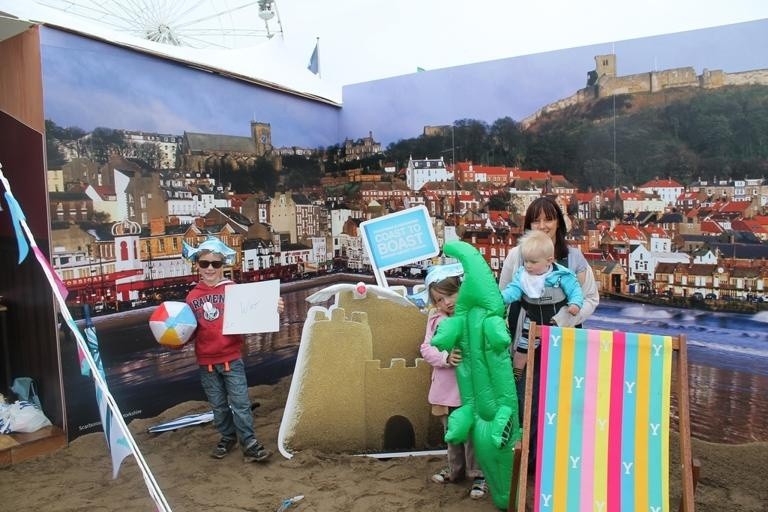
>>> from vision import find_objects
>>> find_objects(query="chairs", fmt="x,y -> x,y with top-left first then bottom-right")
506,319 -> 701,512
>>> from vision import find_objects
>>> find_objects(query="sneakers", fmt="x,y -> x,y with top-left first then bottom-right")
431,471 -> 450,484
470,477 -> 488,500
244,441 -> 273,462
213,434 -> 237,458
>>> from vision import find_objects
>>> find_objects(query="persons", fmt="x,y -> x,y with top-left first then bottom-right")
498,195 -> 600,478
502,228 -> 582,388
180,239 -> 285,465
419,261 -> 487,500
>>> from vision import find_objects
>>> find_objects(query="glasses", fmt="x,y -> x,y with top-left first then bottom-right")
197,259 -> 225,269
